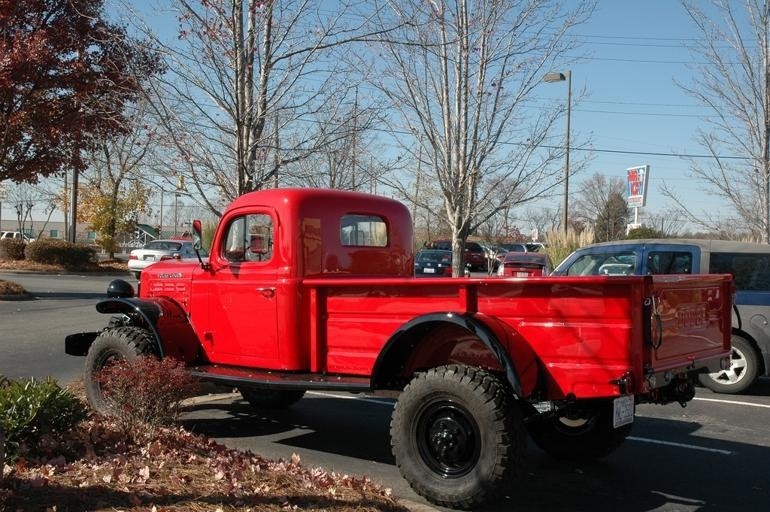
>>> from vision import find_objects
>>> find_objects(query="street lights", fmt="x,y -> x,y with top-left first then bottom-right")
543,68 -> 572,235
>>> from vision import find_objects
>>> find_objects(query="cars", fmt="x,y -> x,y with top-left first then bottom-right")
1,230 -> 35,244
128,240 -> 206,281
411,235 -> 554,279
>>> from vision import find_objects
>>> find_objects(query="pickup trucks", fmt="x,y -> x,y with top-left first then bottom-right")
65,188 -> 732,509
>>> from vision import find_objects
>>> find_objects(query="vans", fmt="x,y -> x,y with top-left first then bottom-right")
552,240 -> 769,394
